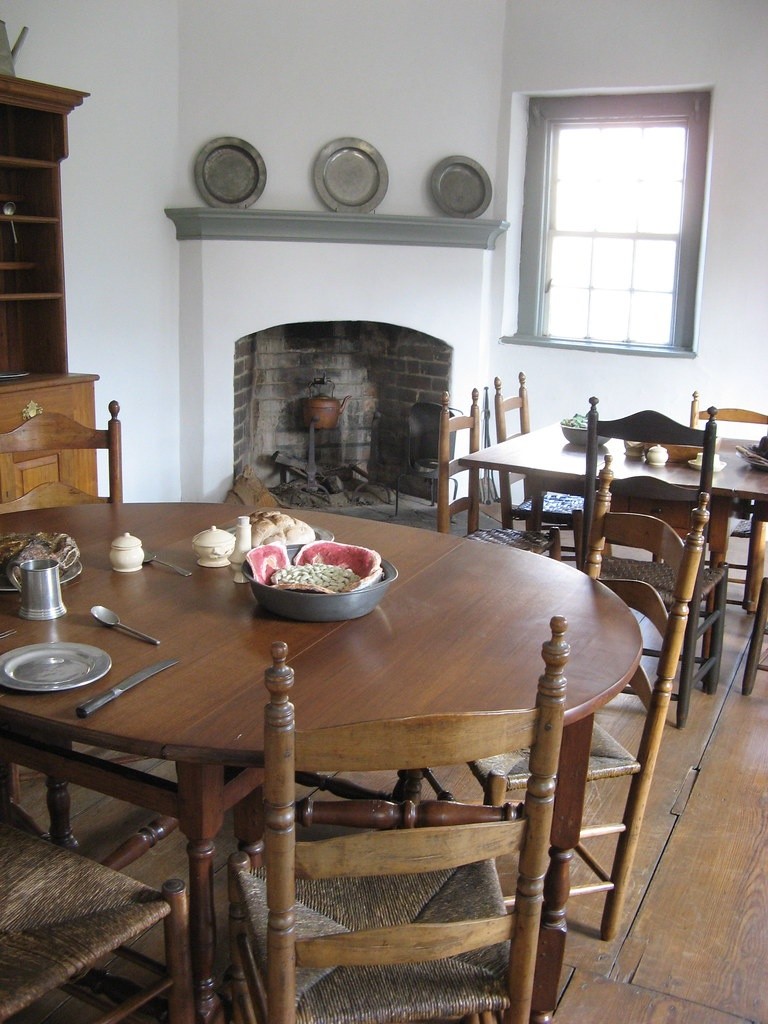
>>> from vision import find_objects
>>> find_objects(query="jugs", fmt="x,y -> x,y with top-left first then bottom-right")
0,19 -> 29,77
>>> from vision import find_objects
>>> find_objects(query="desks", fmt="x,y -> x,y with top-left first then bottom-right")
458,427 -> 768,690
0,504 -> 642,1023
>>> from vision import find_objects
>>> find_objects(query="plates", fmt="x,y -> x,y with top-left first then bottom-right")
314,138 -> 389,213
736,446 -> 768,473
195,136 -> 267,208
0,372 -> 32,380
0,561 -> 82,591
431,155 -> 492,219
226,526 -> 335,545
0,642 -> 112,691
688,460 -> 727,472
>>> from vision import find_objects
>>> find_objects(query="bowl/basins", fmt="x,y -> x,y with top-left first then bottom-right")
624,440 -> 643,457
241,544 -> 398,621
696,452 -> 720,464
561,424 -> 611,446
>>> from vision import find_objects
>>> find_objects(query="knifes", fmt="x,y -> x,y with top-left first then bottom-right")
75,658 -> 180,718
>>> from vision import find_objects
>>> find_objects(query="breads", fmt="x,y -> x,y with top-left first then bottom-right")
248,510 -> 316,547
0,530 -> 81,586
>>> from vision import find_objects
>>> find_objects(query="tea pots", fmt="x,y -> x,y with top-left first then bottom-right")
303,380 -> 352,429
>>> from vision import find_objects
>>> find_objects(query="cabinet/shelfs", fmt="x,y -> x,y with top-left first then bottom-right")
0,77 -> 100,512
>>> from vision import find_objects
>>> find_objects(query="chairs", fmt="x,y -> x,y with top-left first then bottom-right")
0,373 -> 768,1024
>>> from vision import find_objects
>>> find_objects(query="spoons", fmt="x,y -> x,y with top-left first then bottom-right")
2,202 -> 18,243
143,552 -> 192,577
91,606 -> 161,645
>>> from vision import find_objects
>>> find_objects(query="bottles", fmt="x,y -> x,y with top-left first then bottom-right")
228,516 -> 252,564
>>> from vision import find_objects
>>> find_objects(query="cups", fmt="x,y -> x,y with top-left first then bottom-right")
192,526 -> 236,567
7,560 -> 68,620
110,532 -> 145,573
647,444 -> 668,466
